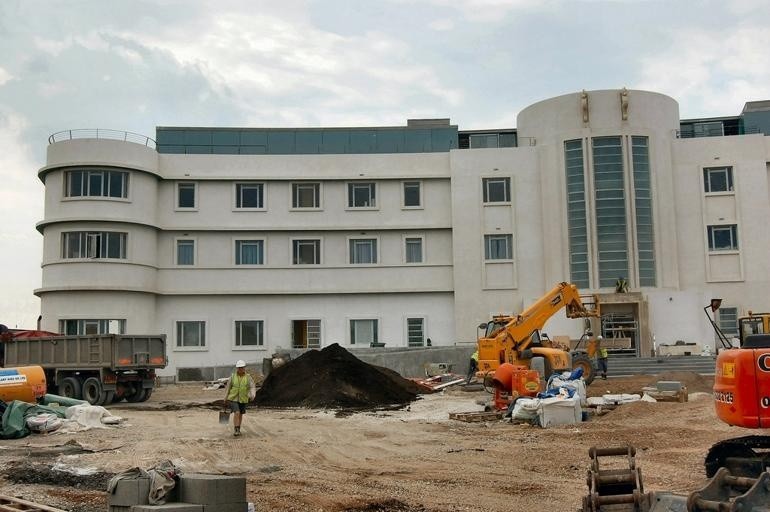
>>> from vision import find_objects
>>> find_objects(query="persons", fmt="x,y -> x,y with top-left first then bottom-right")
595,335 -> 608,381
464,350 -> 479,384
223,359 -> 256,436
614,274 -> 629,294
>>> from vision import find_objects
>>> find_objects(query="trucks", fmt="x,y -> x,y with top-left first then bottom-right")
0,314 -> 167,407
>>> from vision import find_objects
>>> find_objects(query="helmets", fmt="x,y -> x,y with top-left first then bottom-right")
236,360 -> 246,367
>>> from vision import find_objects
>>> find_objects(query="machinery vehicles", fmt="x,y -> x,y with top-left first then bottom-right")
0,365 -> 47,411
473,281 -> 633,395
700,296 -> 770,495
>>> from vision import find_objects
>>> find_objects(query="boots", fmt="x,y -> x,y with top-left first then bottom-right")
234,426 -> 240,436
